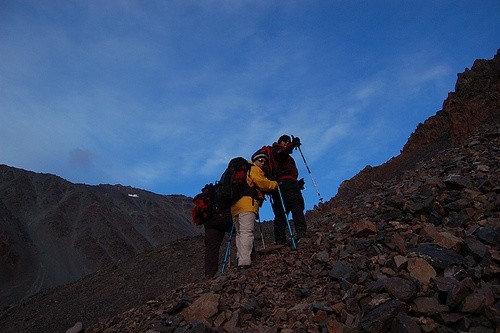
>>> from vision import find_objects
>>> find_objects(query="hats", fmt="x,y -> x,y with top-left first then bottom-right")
278,135 -> 291,143
252,149 -> 268,162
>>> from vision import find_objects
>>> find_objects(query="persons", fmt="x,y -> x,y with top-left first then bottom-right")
204,135 -> 312,276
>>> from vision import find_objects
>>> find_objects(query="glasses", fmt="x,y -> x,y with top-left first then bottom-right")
257,159 -> 266,163
283,139 -> 291,143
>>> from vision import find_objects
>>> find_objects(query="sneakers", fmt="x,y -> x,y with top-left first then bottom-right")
239,265 -> 253,272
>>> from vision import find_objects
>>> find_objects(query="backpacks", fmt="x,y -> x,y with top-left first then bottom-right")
259,143 -> 281,181
230,163 -> 254,205
191,180 -> 221,227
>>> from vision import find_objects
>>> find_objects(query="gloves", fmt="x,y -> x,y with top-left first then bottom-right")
292,137 -> 300,146
